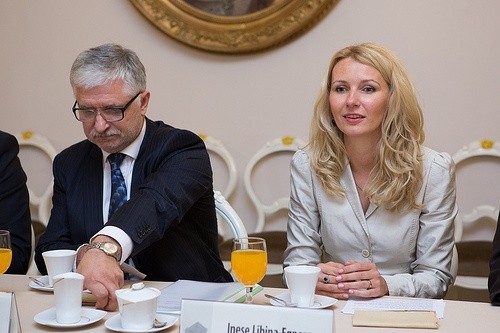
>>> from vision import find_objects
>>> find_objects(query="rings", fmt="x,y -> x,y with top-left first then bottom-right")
323,276 -> 329,283
370,281 -> 371,288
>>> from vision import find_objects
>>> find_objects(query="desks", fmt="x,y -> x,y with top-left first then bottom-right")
1,272 -> 500,332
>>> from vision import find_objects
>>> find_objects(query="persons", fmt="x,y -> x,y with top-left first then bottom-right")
35,44 -> 233,312
0,130 -> 33,276
282,43 -> 457,301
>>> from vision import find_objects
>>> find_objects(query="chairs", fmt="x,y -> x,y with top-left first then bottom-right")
15,131 -> 500,291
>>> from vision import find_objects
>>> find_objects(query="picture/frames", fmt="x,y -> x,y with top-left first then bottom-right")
127,0 -> 341,54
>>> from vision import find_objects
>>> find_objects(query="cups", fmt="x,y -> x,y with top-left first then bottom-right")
52,272 -> 85,324
42,250 -> 79,287
284,266 -> 322,307
115,283 -> 161,330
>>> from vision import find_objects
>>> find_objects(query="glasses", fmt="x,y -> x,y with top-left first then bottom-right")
71,91 -> 141,123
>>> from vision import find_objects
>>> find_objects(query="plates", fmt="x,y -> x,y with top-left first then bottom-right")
29,275 -> 58,291
104,313 -> 179,333
270,293 -> 339,309
34,306 -> 108,328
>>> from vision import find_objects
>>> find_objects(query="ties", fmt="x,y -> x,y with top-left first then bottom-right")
105,151 -> 131,281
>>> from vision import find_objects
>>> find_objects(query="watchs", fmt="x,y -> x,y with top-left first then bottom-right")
86,241 -> 120,261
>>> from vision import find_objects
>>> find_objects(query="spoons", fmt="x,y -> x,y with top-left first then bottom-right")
153,318 -> 167,327
264,294 -> 296,307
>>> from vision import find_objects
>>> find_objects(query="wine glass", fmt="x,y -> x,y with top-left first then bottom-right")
231,237 -> 267,304
0,230 -> 12,291
